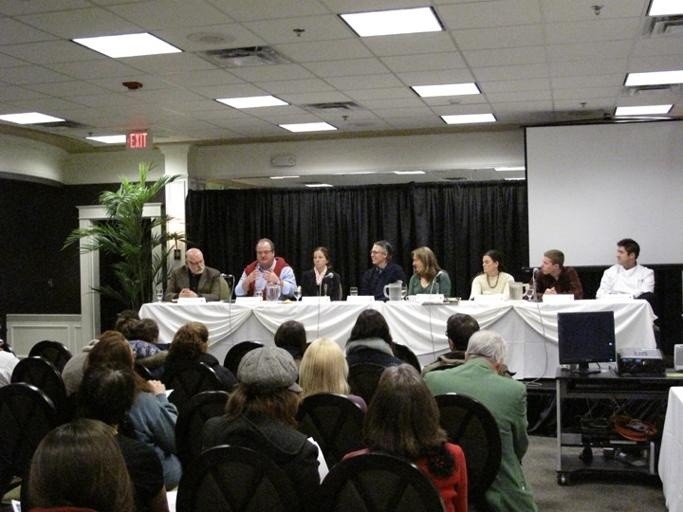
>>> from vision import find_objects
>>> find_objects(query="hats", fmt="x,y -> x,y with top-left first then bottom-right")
237,346 -> 304,394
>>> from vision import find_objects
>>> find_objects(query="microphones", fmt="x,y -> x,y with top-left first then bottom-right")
219,273 -> 232,280
532,267 -> 538,277
322,272 -> 334,281
435,270 -> 443,281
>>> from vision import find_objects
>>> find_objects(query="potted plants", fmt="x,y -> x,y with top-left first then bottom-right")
58,161 -> 193,310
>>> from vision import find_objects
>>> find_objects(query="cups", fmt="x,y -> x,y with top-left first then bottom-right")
155,288 -> 163,303
291,285 -> 302,302
507,280 -> 530,300
350,287 -> 358,296
383,283 -> 402,300
266,284 -> 281,301
526,285 -> 534,300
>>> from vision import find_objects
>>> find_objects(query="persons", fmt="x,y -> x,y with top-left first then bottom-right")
60,331 -> 131,399
596,238 -> 655,302
274,320 -> 308,386
0,347 -> 20,389
126,318 -> 162,360
299,246 -> 340,301
82,331 -> 183,493
72,361 -> 170,512
534,249 -> 584,300
198,347 -> 321,512
27,418 -> 135,512
234,238 -> 297,301
468,249 -> 515,301
426,330 -> 539,512
114,309 -> 139,331
163,247 -> 221,301
361,240 -> 406,301
408,246 -> 451,297
298,335 -> 369,415
154,321 -> 240,393
343,364 -> 468,512
424,313 -> 481,372
345,309 -> 422,408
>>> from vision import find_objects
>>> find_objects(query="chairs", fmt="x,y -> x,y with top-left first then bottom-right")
345,361 -> 385,406
221,274 -> 235,297
160,362 -> 224,411
295,392 -> 365,471
434,392 -> 502,504
173,443 -> 306,512
223,341 -> 263,374
132,361 -> 151,380
175,391 -> 230,466
27,339 -> 73,373
10,356 -> 67,410
314,452 -> 445,512
0,383 -> 60,477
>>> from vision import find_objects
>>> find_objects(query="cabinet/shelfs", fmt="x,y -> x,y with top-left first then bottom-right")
554,367 -> 682,487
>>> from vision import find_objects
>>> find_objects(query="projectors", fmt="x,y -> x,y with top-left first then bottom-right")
617,346 -> 666,377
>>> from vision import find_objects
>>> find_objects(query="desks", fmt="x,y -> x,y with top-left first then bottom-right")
660,386 -> 683,509
139,300 -> 655,378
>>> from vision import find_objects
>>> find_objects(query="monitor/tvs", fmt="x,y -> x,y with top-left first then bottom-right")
556,311 -> 616,376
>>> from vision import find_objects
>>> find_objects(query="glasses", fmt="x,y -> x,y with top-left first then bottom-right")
445,331 -> 452,338
188,259 -> 204,265
256,249 -> 273,254
369,251 -> 386,255
